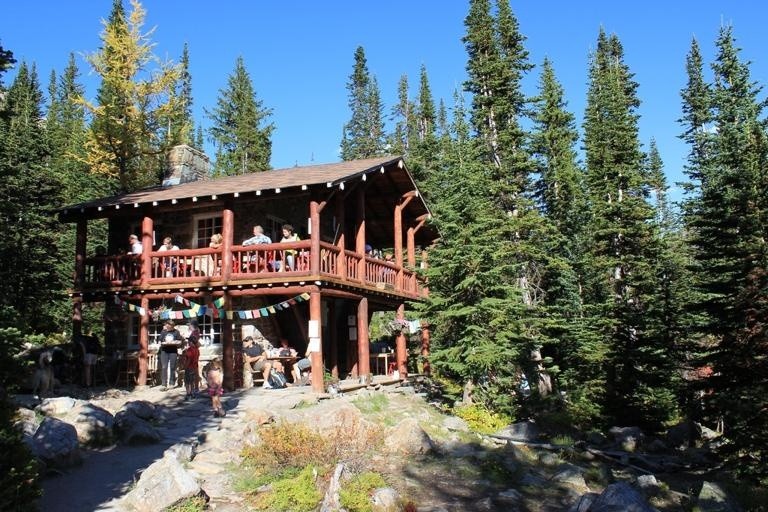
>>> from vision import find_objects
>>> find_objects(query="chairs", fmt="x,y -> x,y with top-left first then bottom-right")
242,354 -> 266,383
107,349 -> 158,388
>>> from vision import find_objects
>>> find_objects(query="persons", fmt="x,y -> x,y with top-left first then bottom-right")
207,358 -> 225,417
365,244 -> 393,263
157,320 -> 184,391
241,334 -> 312,389
95,224 -> 300,281
183,318 -> 200,398
75,331 -> 99,388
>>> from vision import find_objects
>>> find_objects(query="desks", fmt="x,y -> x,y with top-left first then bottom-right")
370,352 -> 395,376
264,355 -> 301,374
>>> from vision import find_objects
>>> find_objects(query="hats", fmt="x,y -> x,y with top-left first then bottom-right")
243,335 -> 254,341
186,336 -> 196,342
